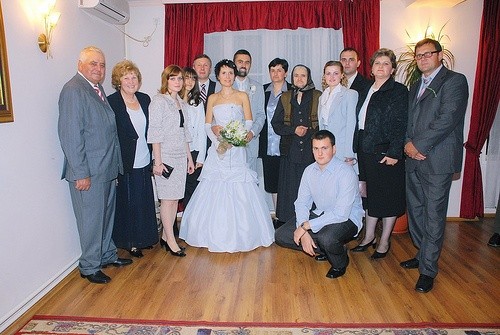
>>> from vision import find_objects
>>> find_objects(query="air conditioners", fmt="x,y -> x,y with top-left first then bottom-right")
77,0 -> 130,25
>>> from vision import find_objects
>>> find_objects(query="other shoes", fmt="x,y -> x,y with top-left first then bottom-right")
129,247 -> 144,258
142,245 -> 153,250
487,233 -> 500,247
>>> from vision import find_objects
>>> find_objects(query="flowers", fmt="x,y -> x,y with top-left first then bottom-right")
216,120 -> 248,153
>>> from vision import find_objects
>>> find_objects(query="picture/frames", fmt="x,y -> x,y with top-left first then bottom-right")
0,0 -> 14,123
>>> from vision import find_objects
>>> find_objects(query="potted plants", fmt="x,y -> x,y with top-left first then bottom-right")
392,17 -> 455,234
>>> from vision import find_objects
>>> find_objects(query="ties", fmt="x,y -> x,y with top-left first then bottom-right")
418,76 -> 432,100
94,84 -> 105,101
199,84 -> 207,104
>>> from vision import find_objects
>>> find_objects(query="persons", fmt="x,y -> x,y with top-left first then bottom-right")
105,60 -> 153,257
354,49 -> 408,260
54,46 -> 133,284
488,197 -> 500,248
273,130 -> 364,278
149,46 -> 369,256
398,38 -> 469,292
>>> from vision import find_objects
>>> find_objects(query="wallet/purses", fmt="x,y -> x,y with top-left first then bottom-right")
149,159 -> 174,178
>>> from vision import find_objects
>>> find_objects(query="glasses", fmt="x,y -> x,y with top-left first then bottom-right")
414,50 -> 438,60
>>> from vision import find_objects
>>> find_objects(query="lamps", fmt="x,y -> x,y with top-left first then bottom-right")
38,0 -> 61,60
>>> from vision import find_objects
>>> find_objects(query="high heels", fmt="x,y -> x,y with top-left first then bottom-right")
165,241 -> 186,256
349,236 -> 377,252
160,237 -> 186,250
371,241 -> 391,258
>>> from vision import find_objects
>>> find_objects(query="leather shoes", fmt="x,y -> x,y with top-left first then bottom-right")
415,274 -> 434,293
80,270 -> 111,284
326,266 -> 346,278
315,254 -> 329,261
399,258 -> 420,269
102,256 -> 133,269
